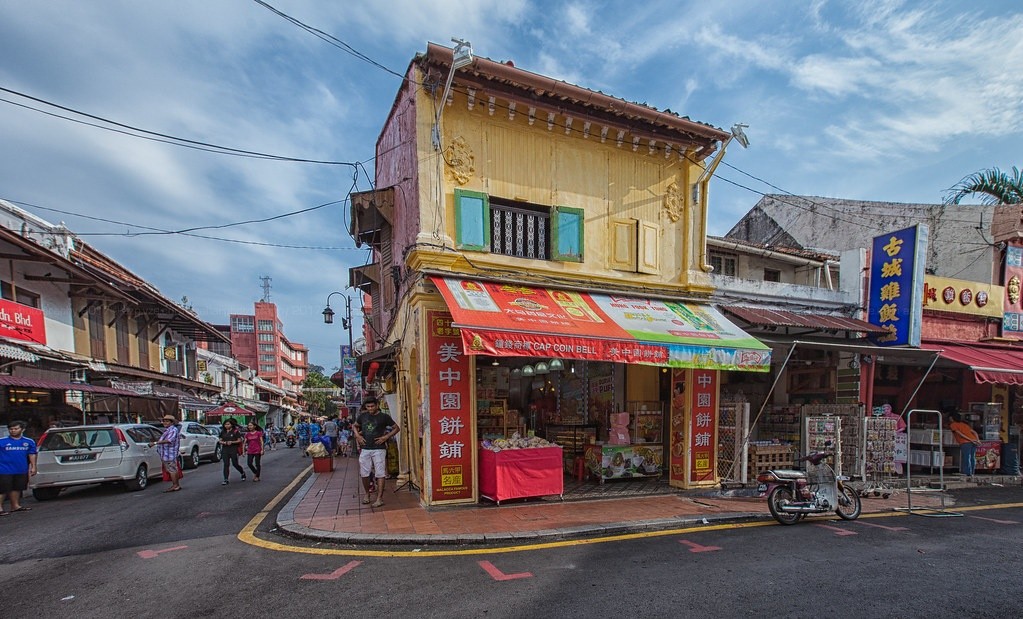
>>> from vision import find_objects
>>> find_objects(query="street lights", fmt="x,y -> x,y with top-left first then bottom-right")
322,291 -> 352,357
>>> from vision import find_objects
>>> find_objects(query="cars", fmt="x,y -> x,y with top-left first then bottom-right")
153,422 -> 223,471
203,424 -> 224,435
28,424 -> 183,500
237,423 -> 299,450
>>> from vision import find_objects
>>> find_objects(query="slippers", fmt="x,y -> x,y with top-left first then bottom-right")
10,505 -> 32,512
0,511 -> 10,516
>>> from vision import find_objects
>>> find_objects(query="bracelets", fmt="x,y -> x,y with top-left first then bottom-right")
154,442 -> 157,444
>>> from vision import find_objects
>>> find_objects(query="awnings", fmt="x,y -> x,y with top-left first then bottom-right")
0,376 -> 139,422
723,337 -> 944,482
431,275 -> 772,374
917,344 -> 1023,386
721,307 -> 889,333
152,386 -> 218,411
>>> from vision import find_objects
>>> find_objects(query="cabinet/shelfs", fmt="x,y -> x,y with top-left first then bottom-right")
475,399 -> 506,439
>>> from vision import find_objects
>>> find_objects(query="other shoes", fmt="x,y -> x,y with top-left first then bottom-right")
241,474 -> 246,481
362,494 -> 370,503
371,499 -> 384,507
222,479 -> 229,485
252,474 -> 260,481
168,484 -> 181,492
369,483 -> 374,493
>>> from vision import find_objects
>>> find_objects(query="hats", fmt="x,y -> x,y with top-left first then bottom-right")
158,415 -> 179,425
364,396 -> 377,403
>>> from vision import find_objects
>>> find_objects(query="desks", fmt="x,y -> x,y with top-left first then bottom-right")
479,444 -> 564,505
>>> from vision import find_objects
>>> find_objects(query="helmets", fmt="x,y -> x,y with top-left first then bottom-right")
290,422 -> 294,426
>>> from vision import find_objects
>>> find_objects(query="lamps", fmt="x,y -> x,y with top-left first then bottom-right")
521,364 -> 535,377
695,123 -> 750,203
534,360 -> 550,375
431,37 -> 472,150
547,358 -> 564,371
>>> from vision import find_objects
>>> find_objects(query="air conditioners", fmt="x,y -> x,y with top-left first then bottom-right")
70,368 -> 86,383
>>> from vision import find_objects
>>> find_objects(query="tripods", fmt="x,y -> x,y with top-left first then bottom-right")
394,376 -> 422,493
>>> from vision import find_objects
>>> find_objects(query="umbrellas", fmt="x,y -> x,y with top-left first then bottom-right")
206,402 -> 256,424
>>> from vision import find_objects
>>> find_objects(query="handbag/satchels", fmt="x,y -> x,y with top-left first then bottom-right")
234,430 -> 242,455
162,461 -> 183,481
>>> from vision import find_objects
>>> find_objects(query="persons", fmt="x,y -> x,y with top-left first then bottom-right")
0,421 -> 37,516
949,414 -> 981,477
149,415 -> 182,492
881,404 -> 906,432
219,419 -> 245,485
287,422 -> 295,432
264,423 -> 277,451
352,396 -> 400,506
242,422 -> 264,481
296,414 -> 354,457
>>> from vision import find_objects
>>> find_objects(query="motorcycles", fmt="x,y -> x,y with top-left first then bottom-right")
283,426 -> 299,448
755,439 -> 863,525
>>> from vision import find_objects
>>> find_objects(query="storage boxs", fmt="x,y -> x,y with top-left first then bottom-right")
909,429 -> 960,467
312,457 -> 333,472
